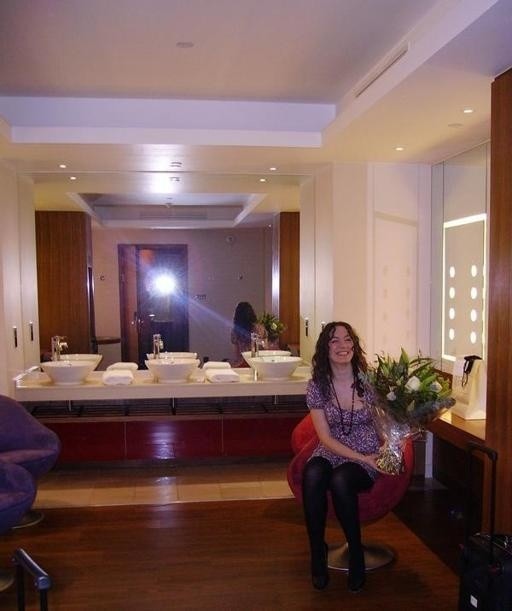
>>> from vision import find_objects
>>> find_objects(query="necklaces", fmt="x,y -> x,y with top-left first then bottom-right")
330,375 -> 359,436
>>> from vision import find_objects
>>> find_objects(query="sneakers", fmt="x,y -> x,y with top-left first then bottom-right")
347,549 -> 366,594
312,541 -> 330,590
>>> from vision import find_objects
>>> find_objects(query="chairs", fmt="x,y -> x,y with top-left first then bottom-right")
286,411 -> 416,573
0,393 -> 61,611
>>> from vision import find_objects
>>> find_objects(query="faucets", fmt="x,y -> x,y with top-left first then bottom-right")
49,335 -> 69,357
152,335 -> 159,357
54,336 -> 62,358
157,333 -> 164,348
251,337 -> 259,358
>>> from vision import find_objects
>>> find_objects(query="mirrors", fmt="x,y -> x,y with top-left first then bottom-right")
16,171 -> 316,374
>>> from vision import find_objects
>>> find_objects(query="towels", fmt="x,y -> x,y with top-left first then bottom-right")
102,369 -> 133,385
202,361 -> 231,368
105,361 -> 139,373
205,368 -> 239,383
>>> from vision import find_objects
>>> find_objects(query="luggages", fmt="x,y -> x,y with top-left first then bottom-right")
457,440 -> 512,610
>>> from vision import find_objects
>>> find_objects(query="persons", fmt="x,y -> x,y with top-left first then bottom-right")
230,300 -> 264,368
299,320 -> 398,594
144,263 -> 178,351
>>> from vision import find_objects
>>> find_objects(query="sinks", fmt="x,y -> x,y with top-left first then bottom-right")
145,359 -> 199,382
241,350 -> 292,373
249,356 -> 302,378
49,353 -> 102,373
147,351 -> 198,358
40,361 -> 94,384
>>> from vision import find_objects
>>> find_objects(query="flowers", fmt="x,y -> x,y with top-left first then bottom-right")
261,309 -> 286,344
358,347 -> 456,477
